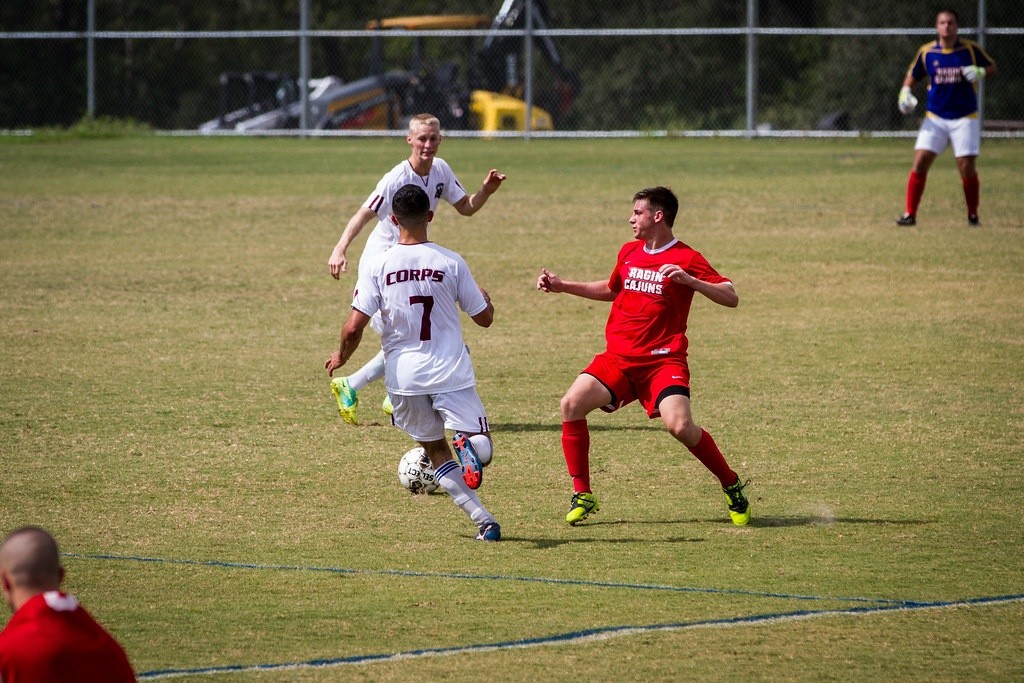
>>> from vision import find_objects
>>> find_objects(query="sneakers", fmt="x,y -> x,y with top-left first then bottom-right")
452,431 -> 482,489
477,523 -> 501,543
567,491 -> 602,526
330,375 -> 362,426
383,395 -> 395,416
721,478 -> 751,527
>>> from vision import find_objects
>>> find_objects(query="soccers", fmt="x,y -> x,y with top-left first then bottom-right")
398,447 -> 440,495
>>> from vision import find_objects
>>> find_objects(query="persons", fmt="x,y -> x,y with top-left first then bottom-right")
0,526 -> 135,683
329,114 -> 507,425
325,184 -> 503,543
895,11 -> 997,226
381,69 -> 410,129
538,186 -> 754,528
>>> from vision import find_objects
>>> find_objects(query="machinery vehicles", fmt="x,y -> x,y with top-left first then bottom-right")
196,1 -> 560,139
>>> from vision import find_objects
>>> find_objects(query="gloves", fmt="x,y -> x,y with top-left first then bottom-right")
897,86 -> 918,115
961,64 -> 986,85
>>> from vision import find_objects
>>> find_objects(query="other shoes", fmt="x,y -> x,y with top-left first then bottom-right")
967,214 -> 980,226
896,211 -> 916,226
